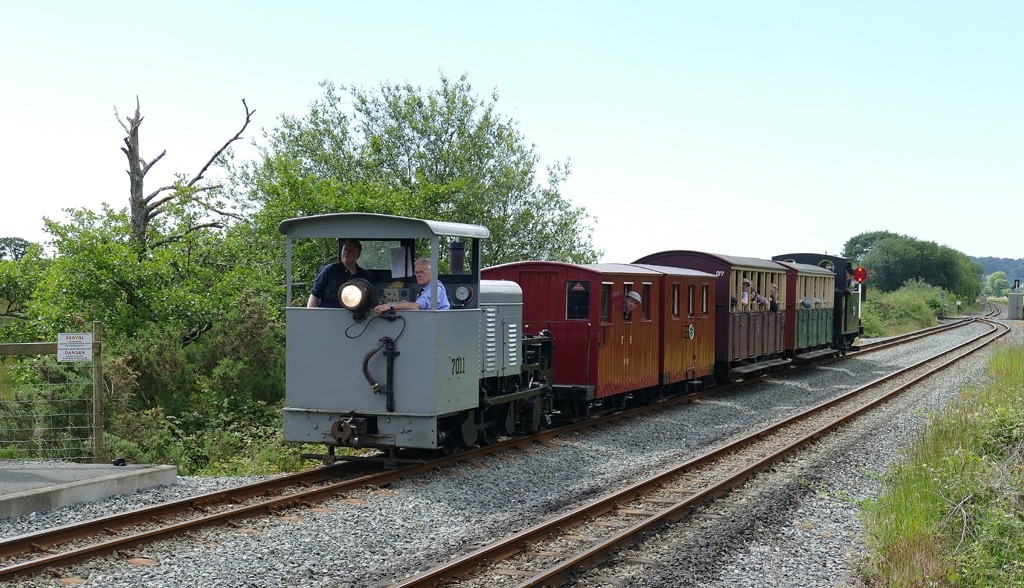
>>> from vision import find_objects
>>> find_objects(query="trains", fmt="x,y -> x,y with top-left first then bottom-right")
277,213 -> 863,472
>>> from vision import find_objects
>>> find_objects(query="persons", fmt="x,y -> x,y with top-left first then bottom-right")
799,297 -> 811,308
814,296 -> 822,304
751,283 -> 778,312
375,258 -> 451,314
623,291 -> 641,320
736,279 -> 751,305
306,240 -> 374,307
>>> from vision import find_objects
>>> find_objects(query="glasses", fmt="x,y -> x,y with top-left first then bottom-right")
413,270 -> 429,275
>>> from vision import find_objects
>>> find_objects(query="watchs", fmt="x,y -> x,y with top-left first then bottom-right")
389,302 -> 394,310
